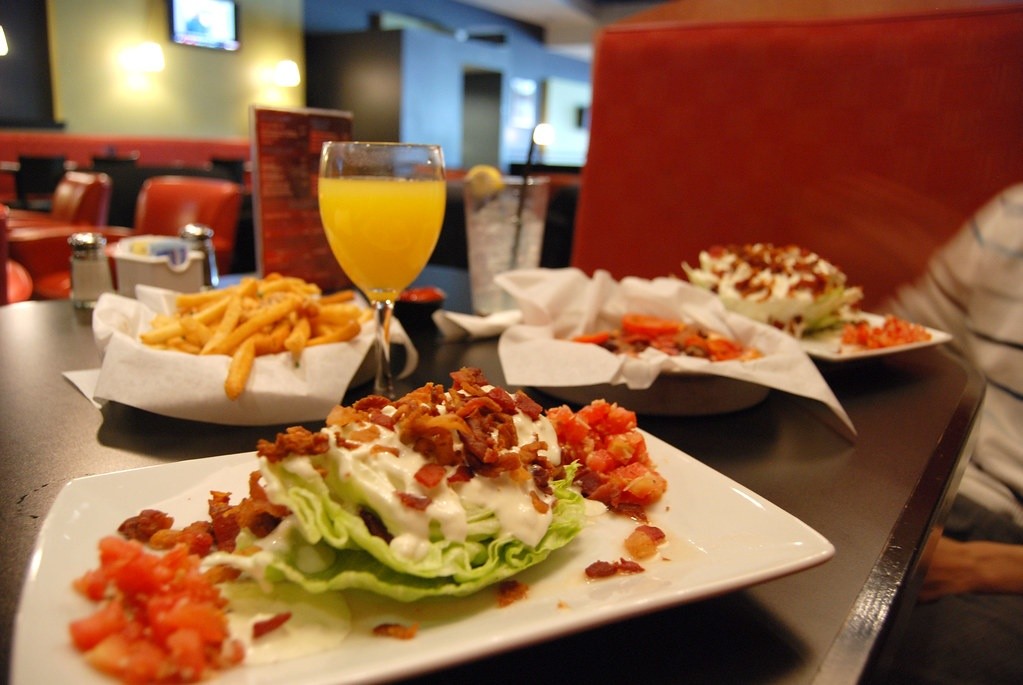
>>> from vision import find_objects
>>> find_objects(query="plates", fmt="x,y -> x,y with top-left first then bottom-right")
794,312 -> 954,360
10,428 -> 833,685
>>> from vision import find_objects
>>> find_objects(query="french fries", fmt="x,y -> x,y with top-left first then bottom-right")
140,271 -> 372,399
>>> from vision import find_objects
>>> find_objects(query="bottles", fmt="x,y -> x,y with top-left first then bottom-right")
181,224 -> 219,287
68,232 -> 113,310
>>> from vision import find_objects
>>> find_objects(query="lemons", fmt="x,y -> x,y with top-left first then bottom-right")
466,165 -> 502,192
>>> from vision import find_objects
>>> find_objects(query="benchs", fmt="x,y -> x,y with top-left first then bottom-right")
573,0 -> 1023,312
8,170 -> 242,303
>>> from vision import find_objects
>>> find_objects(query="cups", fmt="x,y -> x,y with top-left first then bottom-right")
465,176 -> 547,315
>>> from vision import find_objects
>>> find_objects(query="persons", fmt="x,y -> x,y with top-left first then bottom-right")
864,183 -> 1023,685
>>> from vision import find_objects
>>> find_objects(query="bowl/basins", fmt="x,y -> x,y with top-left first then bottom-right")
111,236 -> 204,297
538,372 -> 770,413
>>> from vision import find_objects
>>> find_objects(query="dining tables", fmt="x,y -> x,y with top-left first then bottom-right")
0,264 -> 986,685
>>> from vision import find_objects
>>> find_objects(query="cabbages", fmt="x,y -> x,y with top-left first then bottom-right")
684,250 -> 865,331
210,424 -> 589,601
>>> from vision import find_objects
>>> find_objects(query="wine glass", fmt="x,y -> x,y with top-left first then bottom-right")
318,140 -> 445,403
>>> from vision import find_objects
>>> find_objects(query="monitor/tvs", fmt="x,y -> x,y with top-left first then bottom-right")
169,0 -> 240,51
